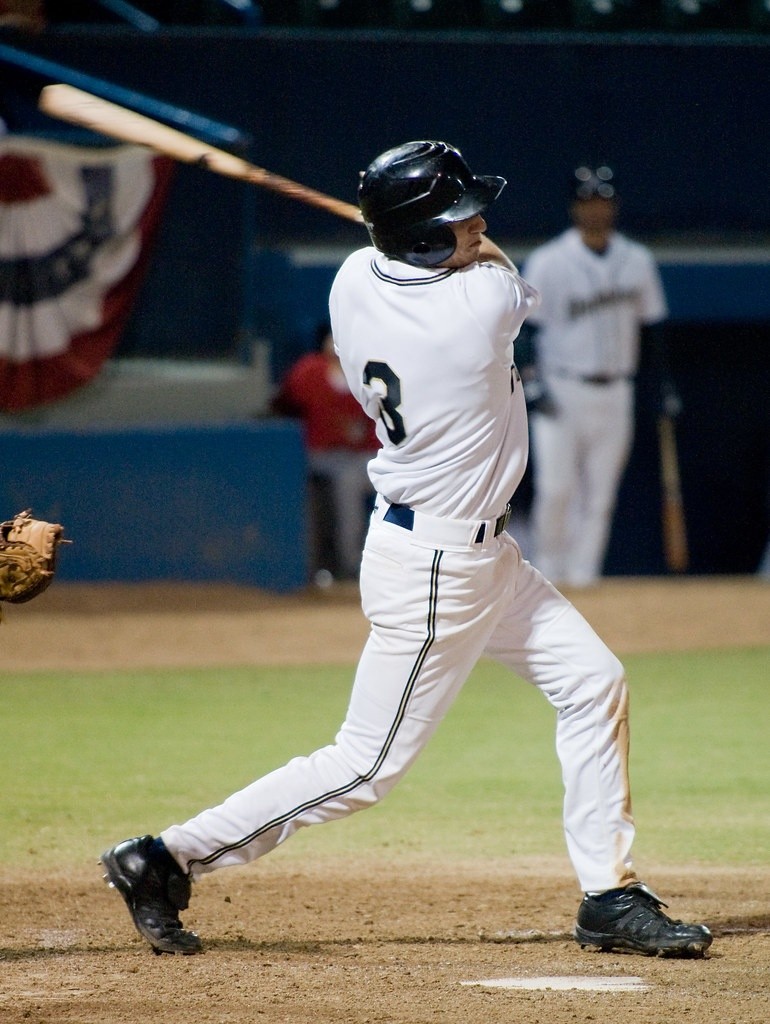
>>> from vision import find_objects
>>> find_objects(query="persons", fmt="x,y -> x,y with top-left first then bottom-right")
271,320 -> 384,576
520,162 -> 681,589
98,141 -> 713,955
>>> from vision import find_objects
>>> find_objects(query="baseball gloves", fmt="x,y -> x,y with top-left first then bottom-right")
0,514 -> 64,605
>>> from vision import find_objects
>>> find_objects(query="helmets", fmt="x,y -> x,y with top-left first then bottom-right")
571,158 -> 624,204
358,139 -> 507,266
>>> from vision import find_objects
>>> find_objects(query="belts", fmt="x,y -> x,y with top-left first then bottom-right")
588,374 -> 613,385
384,501 -> 513,544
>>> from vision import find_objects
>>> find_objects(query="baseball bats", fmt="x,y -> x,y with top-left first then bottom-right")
37,81 -> 369,223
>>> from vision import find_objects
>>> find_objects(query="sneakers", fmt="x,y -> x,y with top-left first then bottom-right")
575,882 -> 714,957
98,834 -> 203,953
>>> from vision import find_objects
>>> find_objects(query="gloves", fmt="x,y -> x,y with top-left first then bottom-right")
523,376 -> 552,417
